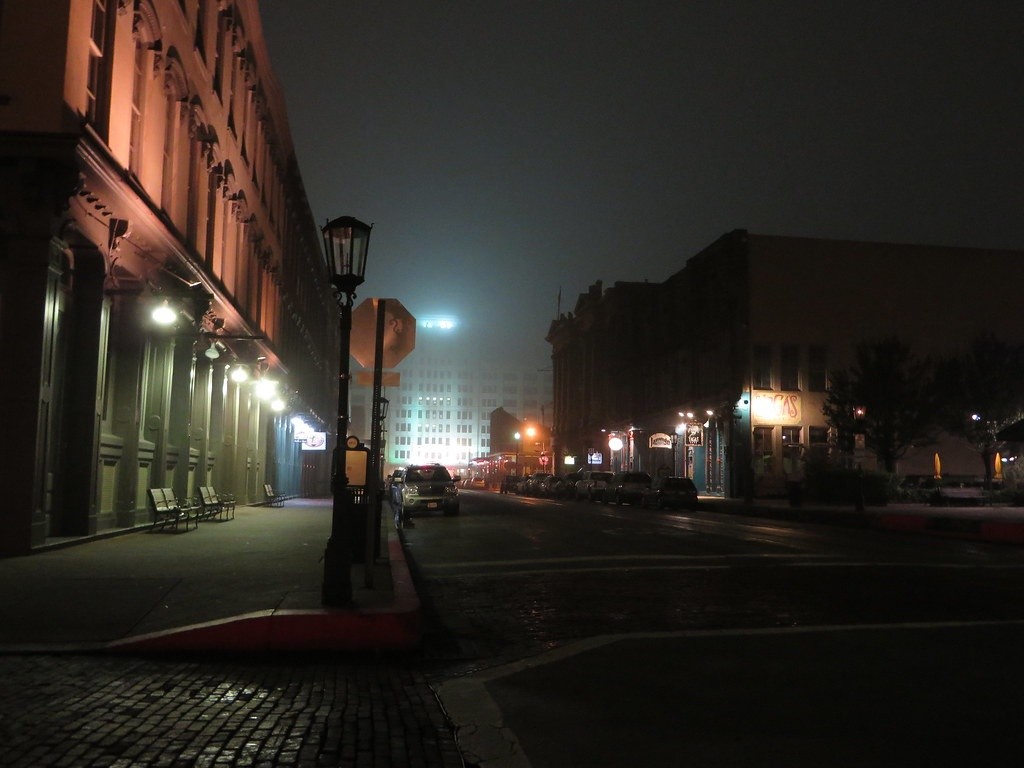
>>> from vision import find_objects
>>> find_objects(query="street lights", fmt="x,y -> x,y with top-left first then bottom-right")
319,212 -> 376,607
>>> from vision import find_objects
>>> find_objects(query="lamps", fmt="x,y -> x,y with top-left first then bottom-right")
149,299 -> 179,328
206,339 -> 220,359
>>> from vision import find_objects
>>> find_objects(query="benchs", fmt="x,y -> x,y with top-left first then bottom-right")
147,487 -> 201,534
937,486 -> 993,507
263,484 -> 286,507
197,486 -> 237,522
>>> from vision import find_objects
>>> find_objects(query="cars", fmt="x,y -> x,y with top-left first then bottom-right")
498,470 -> 699,513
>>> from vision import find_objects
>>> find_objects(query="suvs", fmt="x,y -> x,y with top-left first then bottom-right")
384,461 -> 462,518
